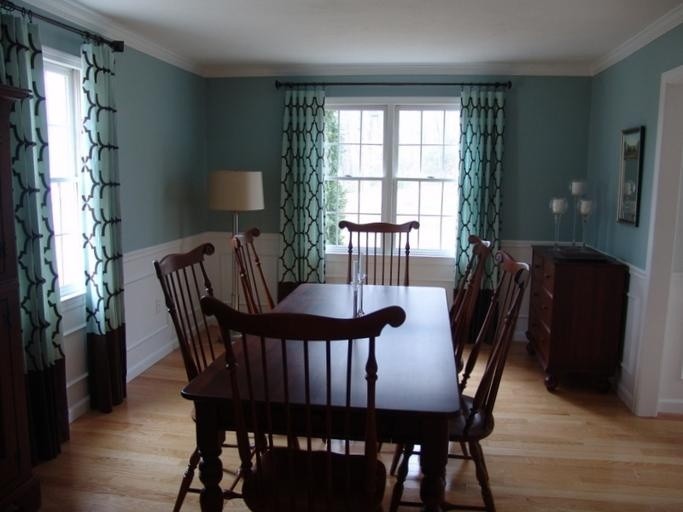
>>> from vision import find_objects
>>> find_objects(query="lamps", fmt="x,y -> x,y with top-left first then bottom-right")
207,169 -> 266,343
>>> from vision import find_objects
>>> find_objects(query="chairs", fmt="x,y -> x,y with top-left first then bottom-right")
386,249 -> 536,511
200,292 -> 407,512
448,232 -> 491,375
335,218 -> 421,287
152,240 -> 264,511
230,228 -> 279,325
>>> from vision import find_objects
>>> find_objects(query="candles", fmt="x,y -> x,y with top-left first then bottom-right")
551,198 -> 567,214
571,182 -> 584,196
580,199 -> 593,214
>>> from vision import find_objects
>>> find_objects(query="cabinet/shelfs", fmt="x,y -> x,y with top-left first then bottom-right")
525,245 -> 630,393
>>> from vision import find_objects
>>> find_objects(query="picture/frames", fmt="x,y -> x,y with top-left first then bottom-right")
616,125 -> 646,228
